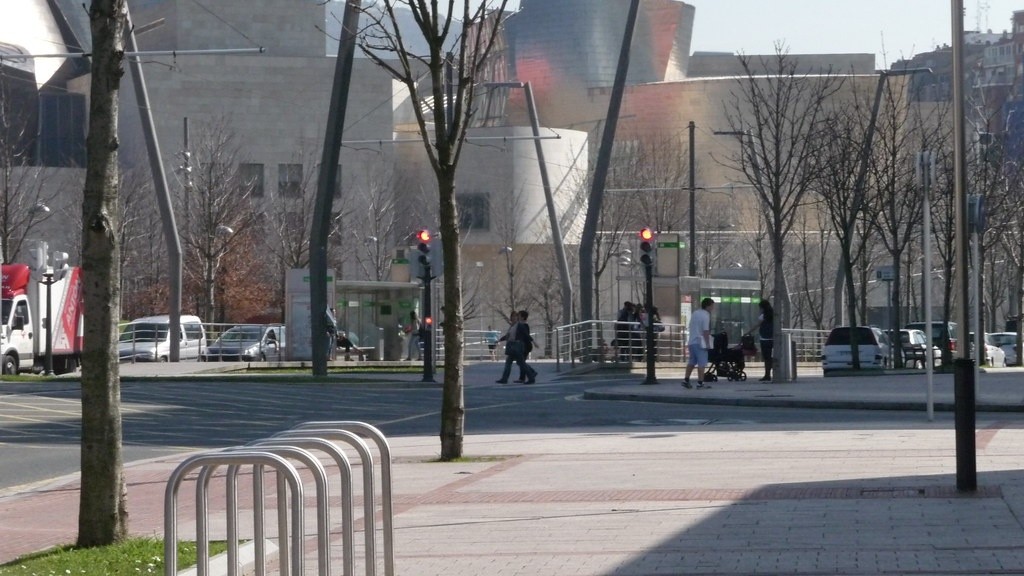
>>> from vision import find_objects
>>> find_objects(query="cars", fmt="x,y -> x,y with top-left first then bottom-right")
968,332 -> 1007,366
821,325 -> 905,373
989,332 -> 1017,364
882,328 -> 941,368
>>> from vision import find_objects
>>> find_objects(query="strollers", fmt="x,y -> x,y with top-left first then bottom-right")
703,331 -> 752,381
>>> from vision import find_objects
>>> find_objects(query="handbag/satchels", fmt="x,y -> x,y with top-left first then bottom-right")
505,339 -> 525,357
652,321 -> 664,332
419,324 -> 424,338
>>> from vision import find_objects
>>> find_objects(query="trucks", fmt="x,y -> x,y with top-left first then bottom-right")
0,264 -> 84,375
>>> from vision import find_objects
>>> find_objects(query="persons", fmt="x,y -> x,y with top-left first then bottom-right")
681,298 -> 715,389
327,308 -> 364,361
495,310 -> 539,384
404,311 -> 422,361
743,300 -> 773,380
614,301 -> 665,361
268,339 -> 279,351
484,325 -> 500,361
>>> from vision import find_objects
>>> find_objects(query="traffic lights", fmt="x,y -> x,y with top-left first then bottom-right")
639,226 -> 655,265
29,247 -> 44,280
53,250 -> 68,280
416,229 -> 431,264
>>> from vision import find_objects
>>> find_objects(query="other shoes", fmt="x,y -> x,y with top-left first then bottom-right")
496,378 -> 507,384
529,372 -> 537,379
524,380 -> 535,385
513,380 -> 524,383
742,333 -> 757,355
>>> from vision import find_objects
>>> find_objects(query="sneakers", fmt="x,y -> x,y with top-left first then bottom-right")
682,380 -> 693,389
759,376 -> 771,381
696,381 -> 712,389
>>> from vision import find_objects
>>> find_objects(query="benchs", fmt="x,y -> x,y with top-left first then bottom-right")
336,346 -> 375,361
903,347 -> 926,369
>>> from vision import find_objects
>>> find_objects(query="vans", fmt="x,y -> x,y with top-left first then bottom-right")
905,321 -> 959,353
119,315 -> 208,363
208,323 -> 286,362
